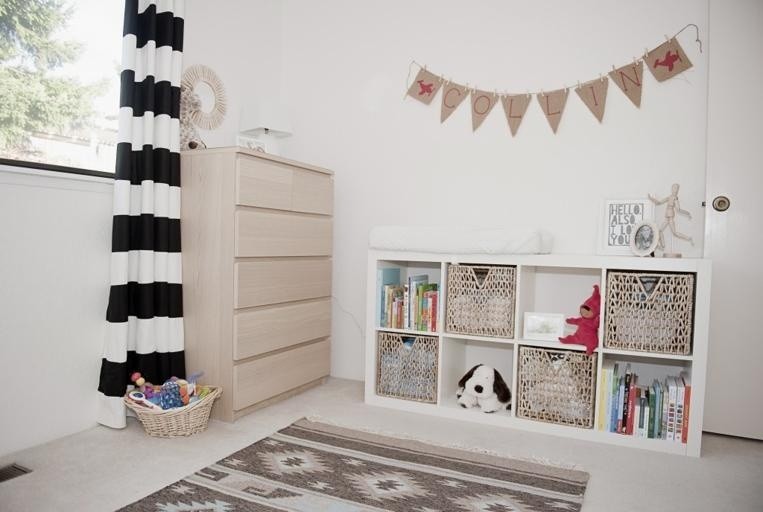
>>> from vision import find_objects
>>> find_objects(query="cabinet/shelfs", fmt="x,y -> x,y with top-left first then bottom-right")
361,248 -> 714,459
179,145 -> 335,425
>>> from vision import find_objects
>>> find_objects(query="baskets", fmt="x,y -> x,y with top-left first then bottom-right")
117,382 -> 220,439
515,342 -> 598,432
443,262 -> 517,340
375,331 -> 439,406
601,269 -> 696,358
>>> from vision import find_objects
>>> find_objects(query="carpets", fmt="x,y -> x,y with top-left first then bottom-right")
108,417 -> 590,512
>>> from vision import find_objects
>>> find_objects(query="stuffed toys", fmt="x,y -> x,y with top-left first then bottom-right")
557,282 -> 599,356
456,361 -> 512,414
127,371 -> 211,412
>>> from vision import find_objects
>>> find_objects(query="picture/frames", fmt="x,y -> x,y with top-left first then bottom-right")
629,221 -> 660,257
606,199 -> 653,256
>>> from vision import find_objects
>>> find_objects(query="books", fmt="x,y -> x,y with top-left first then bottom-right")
597,357 -> 690,442
375,270 -> 437,334
